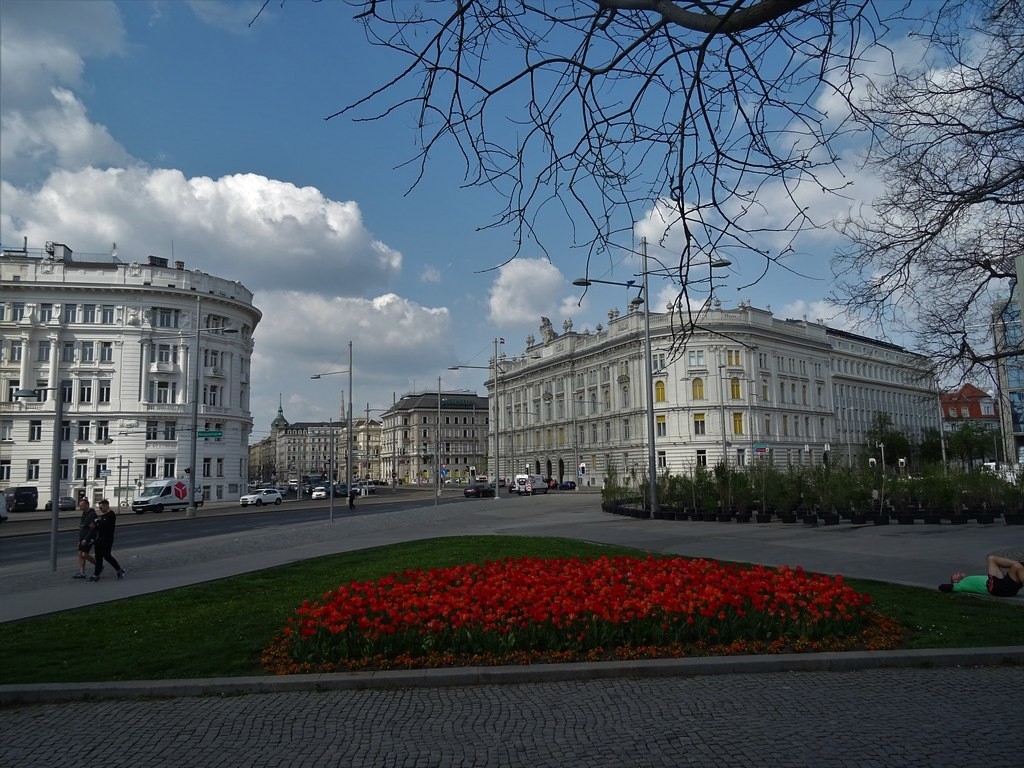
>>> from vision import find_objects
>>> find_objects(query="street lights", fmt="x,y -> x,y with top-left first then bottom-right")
137,296 -> 239,517
364,391 -> 396,494
309,417 -> 334,525
403,376 -> 470,497
13,381 -> 63,573
310,340 -> 353,506
572,237 -> 733,522
544,393 -> 604,492
446,337 -> 542,500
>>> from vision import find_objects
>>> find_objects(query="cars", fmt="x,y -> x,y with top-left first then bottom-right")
445,477 -> 460,484
45,496 -> 76,512
248,478 -> 375,500
312,486 -> 327,501
508,481 -> 516,494
565,481 -> 576,490
490,479 -> 505,488
464,484 -> 495,498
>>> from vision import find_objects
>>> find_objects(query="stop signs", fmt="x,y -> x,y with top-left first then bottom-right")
366,475 -> 370,479
392,473 -> 397,478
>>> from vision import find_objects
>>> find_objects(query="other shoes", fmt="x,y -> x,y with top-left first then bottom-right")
89,574 -> 100,581
73,572 -> 86,578
116,569 -> 126,579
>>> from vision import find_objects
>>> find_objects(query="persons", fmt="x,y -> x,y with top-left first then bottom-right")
542,476 -> 555,488
349,490 -> 355,509
71,500 -> 98,578
938,555 -> 1024,597
88,500 -> 126,582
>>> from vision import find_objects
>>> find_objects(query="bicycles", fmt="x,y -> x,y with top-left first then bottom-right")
120,498 -> 135,509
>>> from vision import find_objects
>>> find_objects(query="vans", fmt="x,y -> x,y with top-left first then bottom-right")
3,486 -> 38,513
981,463 -> 1016,486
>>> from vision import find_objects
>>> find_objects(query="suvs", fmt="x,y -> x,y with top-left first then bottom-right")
240,489 -> 283,508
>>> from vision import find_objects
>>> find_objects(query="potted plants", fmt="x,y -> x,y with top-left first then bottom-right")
602,453 -> 1024,526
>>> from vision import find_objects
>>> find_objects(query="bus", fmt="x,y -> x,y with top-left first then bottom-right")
301,475 -> 322,496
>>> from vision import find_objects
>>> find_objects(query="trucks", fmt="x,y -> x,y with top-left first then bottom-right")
131,478 -> 204,514
515,474 -> 549,496
